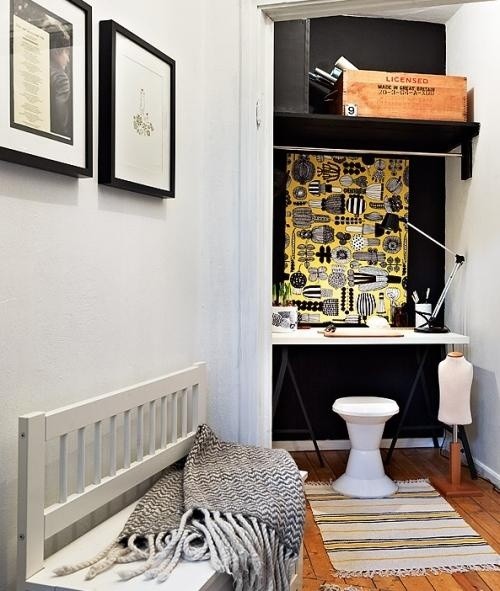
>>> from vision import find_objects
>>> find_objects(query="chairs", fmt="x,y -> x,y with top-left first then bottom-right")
16,360 -> 312,591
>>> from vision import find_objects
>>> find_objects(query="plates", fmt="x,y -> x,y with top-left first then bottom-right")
318,328 -> 404,339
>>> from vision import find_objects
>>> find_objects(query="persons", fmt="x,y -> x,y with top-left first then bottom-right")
41,20 -> 71,138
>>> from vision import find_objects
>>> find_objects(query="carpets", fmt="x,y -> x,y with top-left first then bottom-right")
303,479 -> 500,580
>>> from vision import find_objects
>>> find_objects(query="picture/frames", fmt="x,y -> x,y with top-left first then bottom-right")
0,0 -> 96,181
96,18 -> 179,200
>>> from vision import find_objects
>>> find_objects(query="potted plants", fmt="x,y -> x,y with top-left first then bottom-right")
273,280 -> 301,334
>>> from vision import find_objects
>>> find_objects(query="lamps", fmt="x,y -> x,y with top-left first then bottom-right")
382,212 -> 466,337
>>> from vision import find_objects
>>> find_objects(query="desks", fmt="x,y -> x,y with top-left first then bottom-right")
273,328 -> 480,480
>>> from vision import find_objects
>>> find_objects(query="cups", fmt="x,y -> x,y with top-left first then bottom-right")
273,306 -> 301,329
414,303 -> 432,329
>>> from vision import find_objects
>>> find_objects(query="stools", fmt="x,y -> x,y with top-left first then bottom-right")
332,396 -> 400,499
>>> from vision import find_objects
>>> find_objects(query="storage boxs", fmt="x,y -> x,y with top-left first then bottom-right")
338,69 -> 470,124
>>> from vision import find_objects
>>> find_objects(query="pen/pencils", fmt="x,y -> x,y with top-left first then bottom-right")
411,288 -> 430,304
